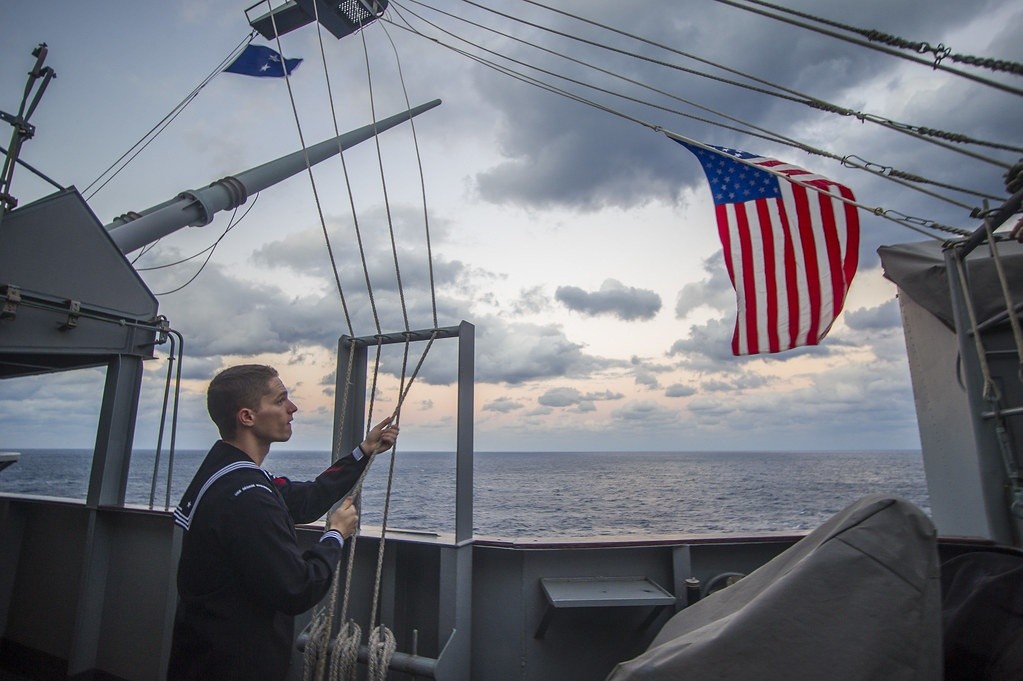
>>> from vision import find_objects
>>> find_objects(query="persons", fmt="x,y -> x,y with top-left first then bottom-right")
166,364 -> 399,681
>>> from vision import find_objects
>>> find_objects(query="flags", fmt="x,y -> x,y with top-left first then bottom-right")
223,43 -> 302,78
668,135 -> 860,356
